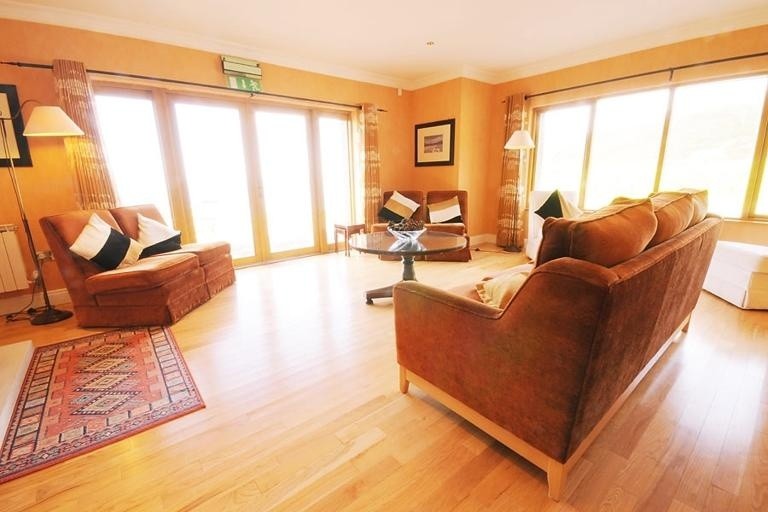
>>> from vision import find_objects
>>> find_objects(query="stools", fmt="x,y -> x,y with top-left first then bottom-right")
335,223 -> 367,256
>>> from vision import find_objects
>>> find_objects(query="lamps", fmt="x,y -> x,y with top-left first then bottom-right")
503,129 -> 536,252
0,100 -> 86,325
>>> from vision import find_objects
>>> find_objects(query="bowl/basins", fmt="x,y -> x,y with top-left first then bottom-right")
387,224 -> 428,241
387,241 -> 426,252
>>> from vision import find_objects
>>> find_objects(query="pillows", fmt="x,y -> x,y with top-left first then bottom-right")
476,271 -> 532,310
533,188 -> 583,220
69,212 -> 144,271
137,212 -> 182,261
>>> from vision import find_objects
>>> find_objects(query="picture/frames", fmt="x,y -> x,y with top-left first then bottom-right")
0,83 -> 35,168
415,118 -> 455,168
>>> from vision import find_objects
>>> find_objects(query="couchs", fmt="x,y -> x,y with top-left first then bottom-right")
38,204 -> 237,334
525,190 -> 576,262
393,212 -> 726,504
372,191 -> 425,261
702,240 -> 768,310
425,191 -> 472,262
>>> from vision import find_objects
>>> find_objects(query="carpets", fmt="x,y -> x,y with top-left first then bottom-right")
1,326 -> 207,485
